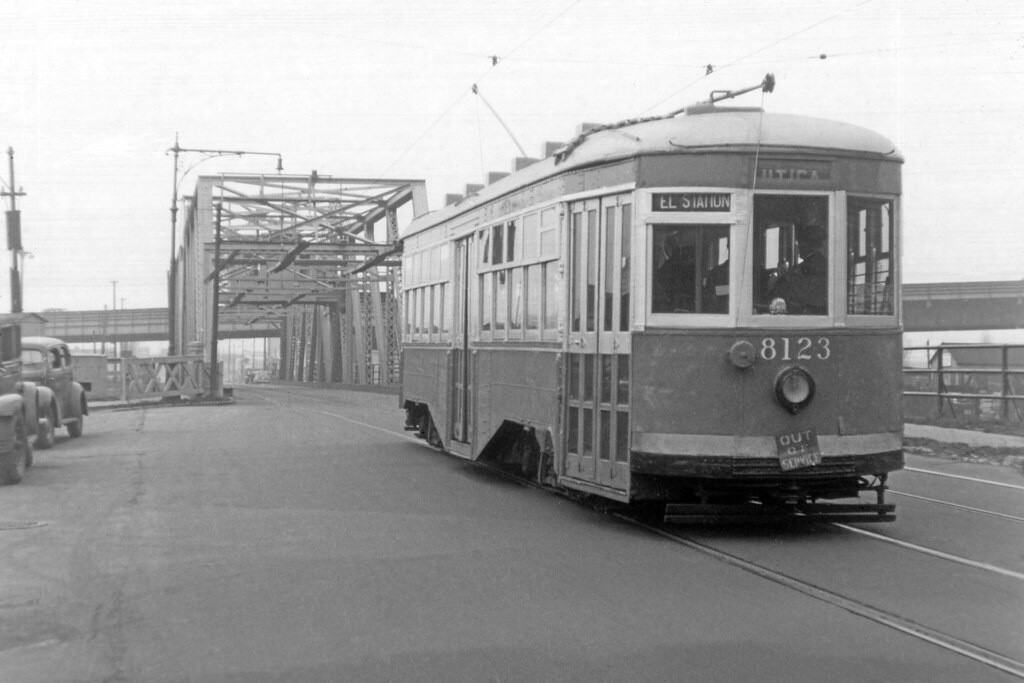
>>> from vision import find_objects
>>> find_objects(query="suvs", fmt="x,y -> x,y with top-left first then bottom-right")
0,308 -> 49,486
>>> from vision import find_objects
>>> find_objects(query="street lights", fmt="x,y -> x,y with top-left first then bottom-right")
17,249 -> 34,298
119,296 -> 126,309
111,280 -> 119,310
165,146 -> 284,398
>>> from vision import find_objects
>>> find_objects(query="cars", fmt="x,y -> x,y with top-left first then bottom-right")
21,331 -> 91,448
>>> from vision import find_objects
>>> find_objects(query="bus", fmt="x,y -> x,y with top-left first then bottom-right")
400,71 -> 904,534
